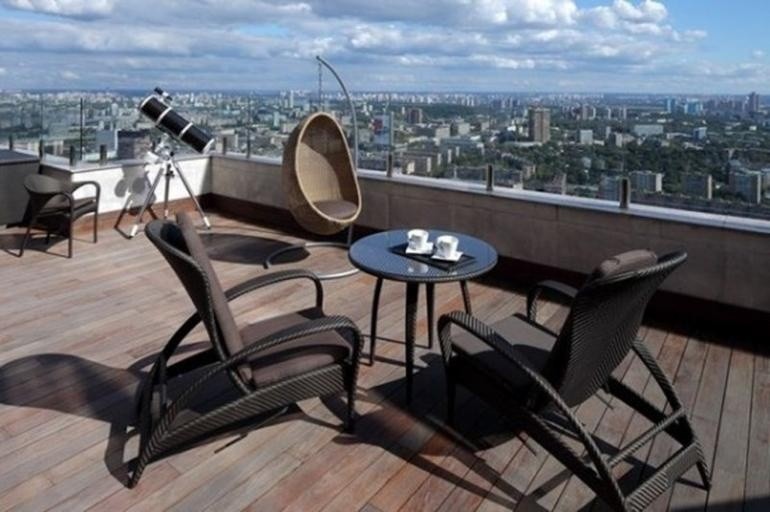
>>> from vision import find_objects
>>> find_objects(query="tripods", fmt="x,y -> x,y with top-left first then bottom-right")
123,133 -> 213,241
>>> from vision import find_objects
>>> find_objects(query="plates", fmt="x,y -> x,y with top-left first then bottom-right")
430,251 -> 463,262
405,242 -> 434,254
388,239 -> 478,273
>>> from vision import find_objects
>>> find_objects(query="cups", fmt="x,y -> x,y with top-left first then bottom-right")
406,229 -> 429,251
436,235 -> 459,258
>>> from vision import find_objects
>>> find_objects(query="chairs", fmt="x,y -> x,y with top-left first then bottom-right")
435,252 -> 712,512
263,57 -> 362,281
126,211 -> 365,488
14,174 -> 100,259
